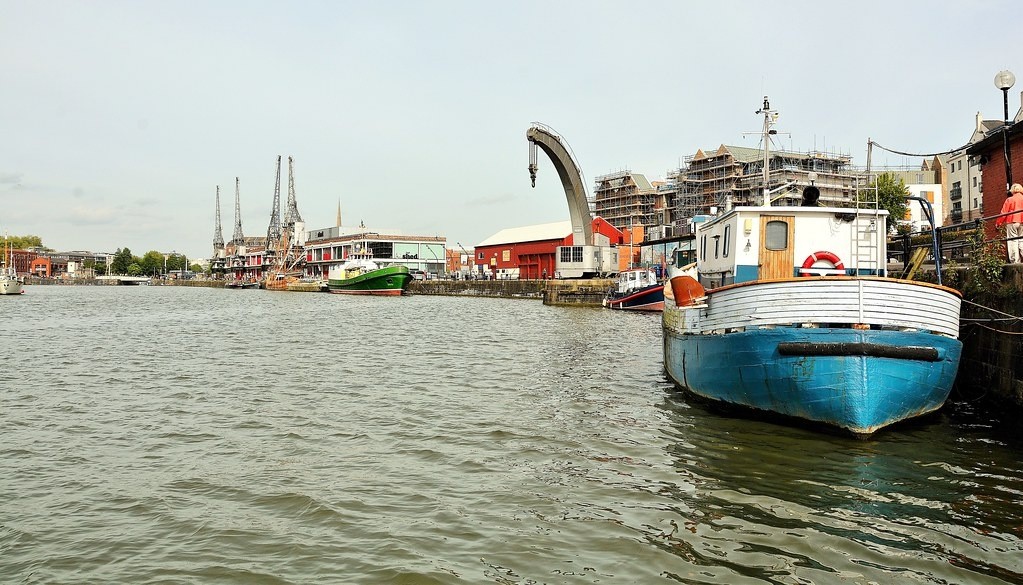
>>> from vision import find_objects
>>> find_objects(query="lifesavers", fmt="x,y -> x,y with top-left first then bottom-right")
801,251 -> 845,276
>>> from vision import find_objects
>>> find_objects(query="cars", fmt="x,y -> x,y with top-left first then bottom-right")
411,267 -> 494,282
648,264 -> 668,277
157,273 -> 226,281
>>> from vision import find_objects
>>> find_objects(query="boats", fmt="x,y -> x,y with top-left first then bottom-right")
0,241 -> 24,296
601,214 -> 666,313
662,94 -> 960,445
324,243 -> 416,297
258,221 -> 329,293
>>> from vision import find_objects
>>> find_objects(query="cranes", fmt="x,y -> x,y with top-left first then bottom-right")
263,154 -> 282,250
210,185 -> 224,258
231,175 -> 246,256
277,154 -> 306,249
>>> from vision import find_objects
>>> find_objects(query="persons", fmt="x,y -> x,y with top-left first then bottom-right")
996,184 -> 1023,264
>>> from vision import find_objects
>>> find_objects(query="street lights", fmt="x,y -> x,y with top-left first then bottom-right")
993,70 -> 1016,199
807,171 -> 818,187
